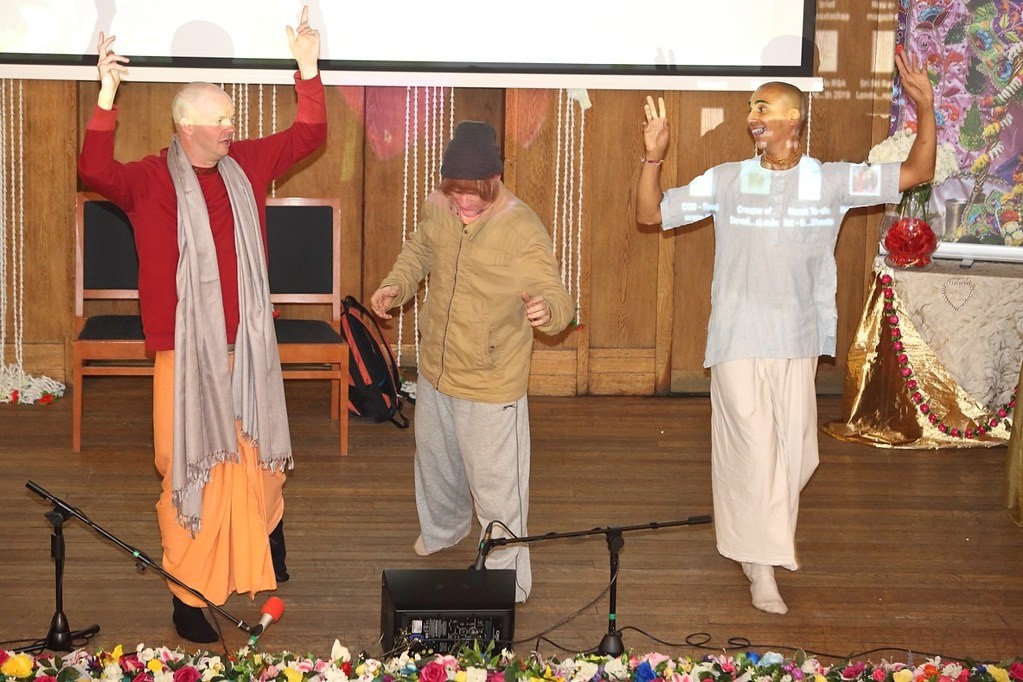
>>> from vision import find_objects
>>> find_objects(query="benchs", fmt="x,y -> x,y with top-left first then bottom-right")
70,190 -> 350,455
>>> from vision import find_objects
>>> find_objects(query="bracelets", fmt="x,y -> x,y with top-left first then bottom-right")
639,155 -> 665,166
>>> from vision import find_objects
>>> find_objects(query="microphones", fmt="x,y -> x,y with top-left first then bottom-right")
244,596 -> 286,652
468,521 -> 494,570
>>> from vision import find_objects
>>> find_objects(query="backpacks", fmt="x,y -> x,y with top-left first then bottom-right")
339,294 -> 410,428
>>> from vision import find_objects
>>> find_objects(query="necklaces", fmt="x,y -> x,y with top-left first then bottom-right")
763,146 -> 802,167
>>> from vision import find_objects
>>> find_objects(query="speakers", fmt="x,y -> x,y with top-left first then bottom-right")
380,568 -> 518,660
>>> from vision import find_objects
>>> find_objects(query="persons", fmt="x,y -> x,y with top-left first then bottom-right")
369,120 -> 577,603
77,5 -> 328,644
635,49 -> 937,615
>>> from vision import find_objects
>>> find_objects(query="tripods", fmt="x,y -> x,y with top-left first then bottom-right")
8,479 -> 250,655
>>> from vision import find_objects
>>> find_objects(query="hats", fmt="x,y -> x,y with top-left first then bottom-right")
440,120 -> 503,181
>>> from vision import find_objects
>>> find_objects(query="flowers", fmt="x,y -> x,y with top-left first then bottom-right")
0,645 -> 1022,682
